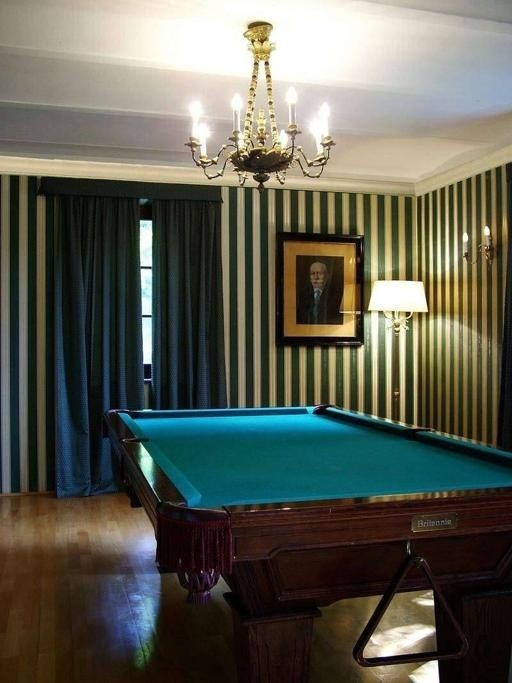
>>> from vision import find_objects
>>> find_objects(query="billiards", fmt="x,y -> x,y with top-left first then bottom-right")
188,569 -> 211,591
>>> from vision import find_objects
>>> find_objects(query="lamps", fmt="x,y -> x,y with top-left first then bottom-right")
368,279 -> 430,418
461,225 -> 496,266
183,22 -> 337,196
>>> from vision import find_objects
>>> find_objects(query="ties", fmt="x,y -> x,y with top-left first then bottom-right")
314,288 -> 321,302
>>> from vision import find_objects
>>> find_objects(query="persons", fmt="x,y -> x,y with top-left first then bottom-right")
296,259 -> 343,324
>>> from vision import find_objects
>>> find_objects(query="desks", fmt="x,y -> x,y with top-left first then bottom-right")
104,403 -> 510,683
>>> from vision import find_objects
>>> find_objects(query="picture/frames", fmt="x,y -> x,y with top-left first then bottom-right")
272,230 -> 364,350
